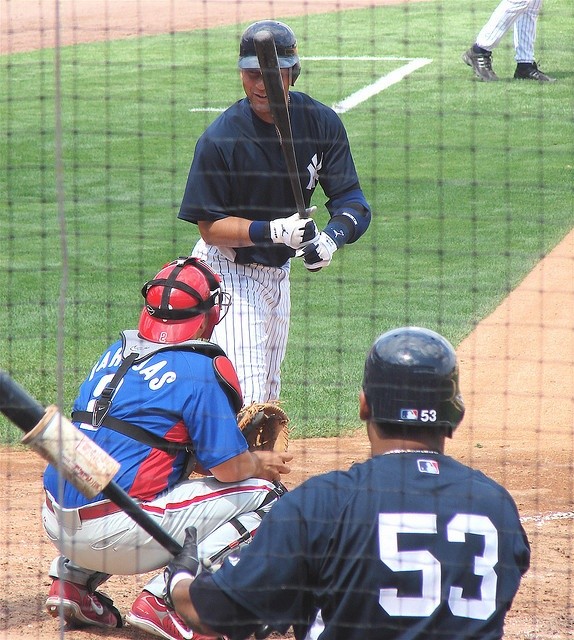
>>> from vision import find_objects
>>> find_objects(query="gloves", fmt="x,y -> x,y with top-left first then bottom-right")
269,206 -> 318,250
296,234 -> 337,271
165,527 -> 212,608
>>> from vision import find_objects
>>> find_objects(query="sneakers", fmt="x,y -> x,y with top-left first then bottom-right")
461,47 -> 498,81
47,580 -> 116,630
514,66 -> 556,82
126,592 -> 222,640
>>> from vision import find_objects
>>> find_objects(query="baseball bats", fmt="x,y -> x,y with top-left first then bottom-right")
1,369 -> 213,573
252,30 -> 324,272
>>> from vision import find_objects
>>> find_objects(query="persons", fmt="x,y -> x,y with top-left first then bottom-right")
162,326 -> 531,640
461,0 -> 557,83
40,255 -> 293,639
178,20 -> 372,409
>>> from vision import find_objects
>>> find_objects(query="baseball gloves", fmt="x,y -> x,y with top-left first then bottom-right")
193,403 -> 290,477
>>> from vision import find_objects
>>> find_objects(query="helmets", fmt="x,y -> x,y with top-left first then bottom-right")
363,329 -> 461,432
138,258 -> 222,343
240,22 -> 298,68
